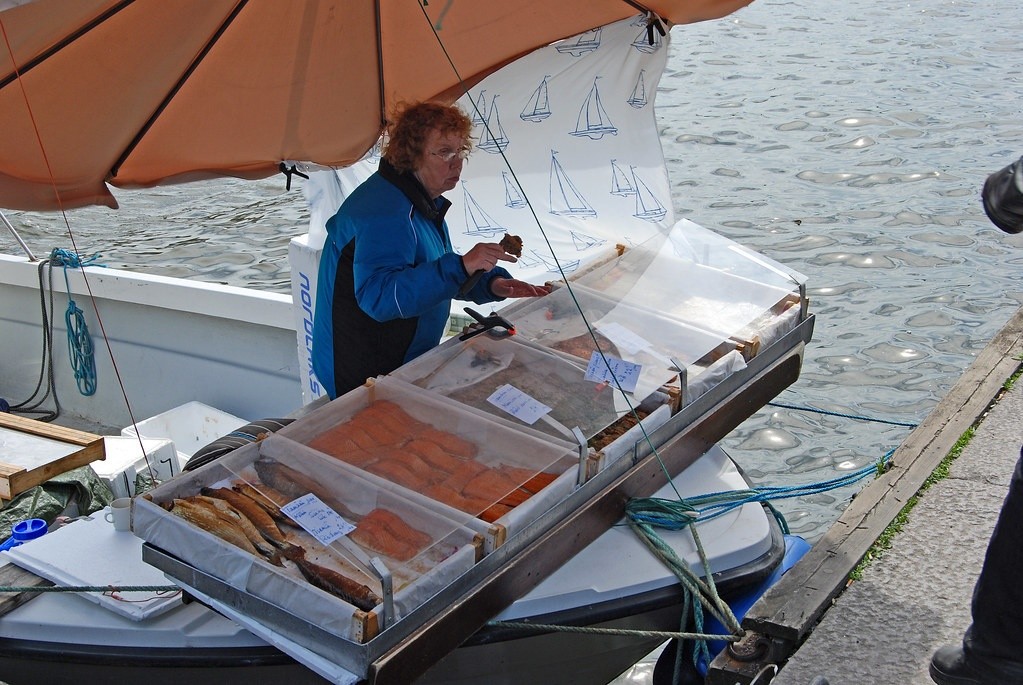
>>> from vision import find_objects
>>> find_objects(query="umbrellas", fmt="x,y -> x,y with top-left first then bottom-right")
0,0 -> 754,212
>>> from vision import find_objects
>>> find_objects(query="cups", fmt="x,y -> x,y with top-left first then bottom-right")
104,497 -> 131,532
12,518 -> 48,547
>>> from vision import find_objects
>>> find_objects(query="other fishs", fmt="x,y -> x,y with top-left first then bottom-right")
159,460 -> 383,611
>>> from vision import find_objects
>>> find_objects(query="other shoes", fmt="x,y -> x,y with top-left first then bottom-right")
928,643 -> 1023,685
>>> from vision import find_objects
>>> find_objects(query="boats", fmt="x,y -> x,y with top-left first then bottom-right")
0,0 -> 815,685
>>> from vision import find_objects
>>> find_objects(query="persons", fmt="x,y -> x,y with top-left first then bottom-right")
930,155 -> 1023,685
311,101 -> 551,401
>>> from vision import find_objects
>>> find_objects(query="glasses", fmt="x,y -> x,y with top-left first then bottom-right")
431,146 -> 469,162
